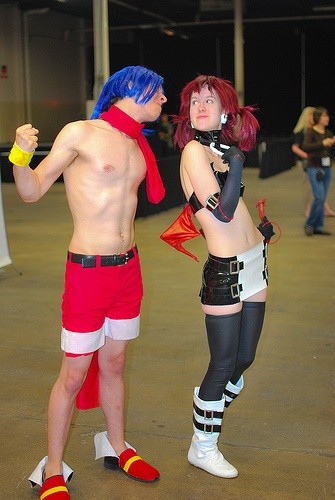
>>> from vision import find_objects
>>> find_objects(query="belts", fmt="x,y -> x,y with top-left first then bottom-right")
65,243 -> 138,268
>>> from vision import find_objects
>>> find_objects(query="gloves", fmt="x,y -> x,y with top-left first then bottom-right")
257,216 -> 277,241
211,145 -> 247,224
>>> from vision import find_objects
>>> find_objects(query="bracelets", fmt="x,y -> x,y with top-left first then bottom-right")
8,141 -> 35,167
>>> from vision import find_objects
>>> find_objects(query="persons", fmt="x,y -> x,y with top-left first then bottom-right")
170,74 -> 274,479
292,106 -> 335,237
8,66 -> 168,500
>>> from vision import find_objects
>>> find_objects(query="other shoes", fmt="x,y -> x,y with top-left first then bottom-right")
314,228 -> 331,236
28,455 -> 74,500
93,429 -> 161,483
304,226 -> 314,236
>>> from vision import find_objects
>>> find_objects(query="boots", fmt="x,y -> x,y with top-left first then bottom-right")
186,385 -> 239,479
224,374 -> 245,410
